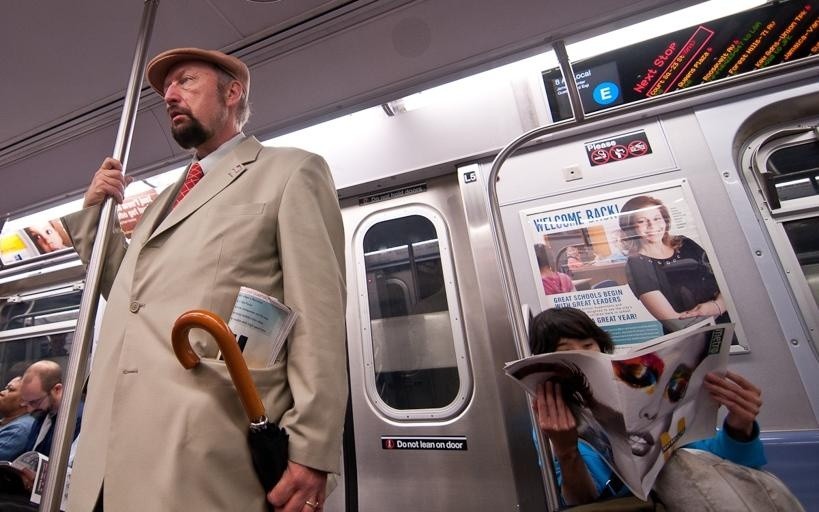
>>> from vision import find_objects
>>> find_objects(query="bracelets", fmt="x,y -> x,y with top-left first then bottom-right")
712,299 -> 724,318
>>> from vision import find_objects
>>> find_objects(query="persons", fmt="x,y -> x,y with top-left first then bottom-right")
24,221 -> 69,255
529,306 -> 763,511
0,357 -> 84,511
619,196 -> 739,345
535,244 -> 585,295
61,47 -> 346,511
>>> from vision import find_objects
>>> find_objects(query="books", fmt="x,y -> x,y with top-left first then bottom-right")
216,285 -> 299,370
504,314 -> 736,500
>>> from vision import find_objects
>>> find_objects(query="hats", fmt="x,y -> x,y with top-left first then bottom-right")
146,47 -> 250,97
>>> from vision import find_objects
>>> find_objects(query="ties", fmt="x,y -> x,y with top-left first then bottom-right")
171,162 -> 204,214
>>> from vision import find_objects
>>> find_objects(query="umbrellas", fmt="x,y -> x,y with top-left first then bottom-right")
170,310 -> 290,511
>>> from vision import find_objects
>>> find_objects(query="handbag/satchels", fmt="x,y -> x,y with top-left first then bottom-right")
654,448 -> 805,512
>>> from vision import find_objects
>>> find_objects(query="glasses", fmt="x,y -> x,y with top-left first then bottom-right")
19,394 -> 46,408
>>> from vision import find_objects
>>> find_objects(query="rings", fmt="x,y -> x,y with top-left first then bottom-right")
305,500 -> 321,509
